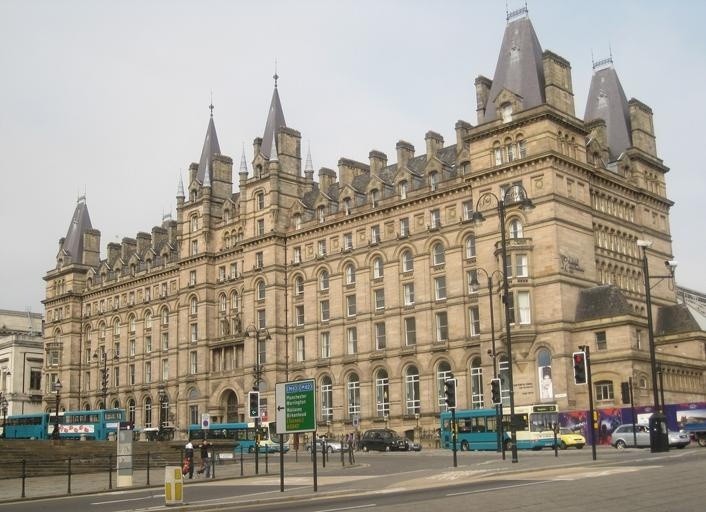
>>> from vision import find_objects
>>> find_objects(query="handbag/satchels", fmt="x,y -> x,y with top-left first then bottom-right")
182,457 -> 190,475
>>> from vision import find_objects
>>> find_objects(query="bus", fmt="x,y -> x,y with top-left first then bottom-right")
187,421 -> 291,454
47,408 -> 127,442
439,402 -> 562,452
0,413 -> 51,441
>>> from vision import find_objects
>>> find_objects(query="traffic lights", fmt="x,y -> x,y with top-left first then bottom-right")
490,377 -> 503,406
444,378 -> 458,410
248,390 -> 260,419
572,351 -> 589,386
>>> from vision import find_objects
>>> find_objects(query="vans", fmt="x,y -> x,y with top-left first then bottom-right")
358,428 -> 399,453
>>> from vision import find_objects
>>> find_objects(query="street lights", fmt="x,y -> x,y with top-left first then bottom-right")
468,182 -> 538,464
244,323 -> 273,424
51,377 -> 64,440
156,384 -> 167,441
467,265 -> 515,452
1,398 -> 9,440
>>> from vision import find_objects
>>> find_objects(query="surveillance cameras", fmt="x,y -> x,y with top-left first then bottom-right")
578,344 -> 585,349
664,261 -> 678,272
637,240 -> 652,247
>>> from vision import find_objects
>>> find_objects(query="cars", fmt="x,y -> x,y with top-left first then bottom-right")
303,437 -> 351,454
557,426 -> 587,450
610,421 -> 692,450
398,435 -> 423,452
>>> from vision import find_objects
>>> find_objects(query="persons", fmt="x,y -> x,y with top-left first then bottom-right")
640,427 -> 645,433
182,438 -> 194,480
196,439 -> 213,479
434,429 -> 441,449
